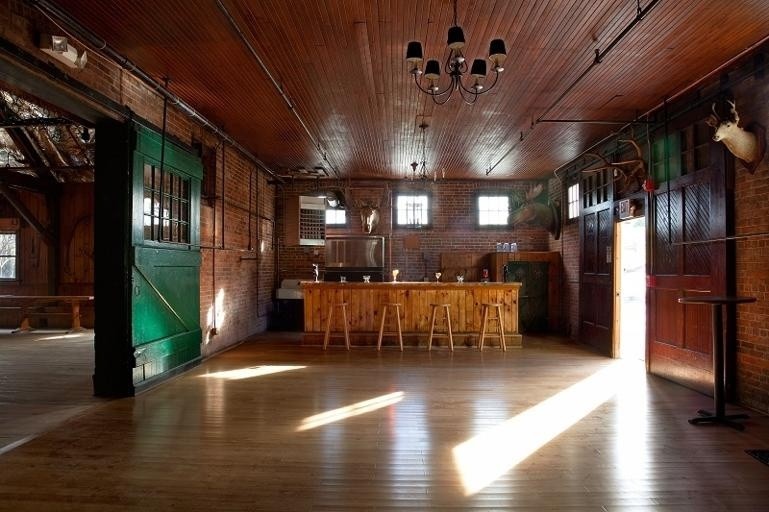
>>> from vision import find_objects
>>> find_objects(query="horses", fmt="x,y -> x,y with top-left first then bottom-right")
352,199 -> 381,234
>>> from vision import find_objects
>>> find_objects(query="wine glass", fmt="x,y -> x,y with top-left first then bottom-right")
363,275 -> 370,284
435,272 -> 441,283
457,275 -> 465,284
392,270 -> 399,283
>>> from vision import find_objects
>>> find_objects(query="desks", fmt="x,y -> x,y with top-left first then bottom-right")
677,295 -> 756,431
0,295 -> 94,334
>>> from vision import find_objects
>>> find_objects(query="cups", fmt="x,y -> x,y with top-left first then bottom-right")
497,242 -> 517,252
341,276 -> 346,283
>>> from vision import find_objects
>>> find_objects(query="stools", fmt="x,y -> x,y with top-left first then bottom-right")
427,303 -> 454,352
323,302 -> 352,351
477,303 -> 507,352
376,303 -> 404,352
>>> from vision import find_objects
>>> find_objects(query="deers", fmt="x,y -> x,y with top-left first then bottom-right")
579,136 -> 644,196
705,99 -> 757,164
507,183 -> 554,233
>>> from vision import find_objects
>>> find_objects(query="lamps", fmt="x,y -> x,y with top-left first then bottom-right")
40,33 -> 88,69
404,124 -> 445,192
406,0 -> 507,105
